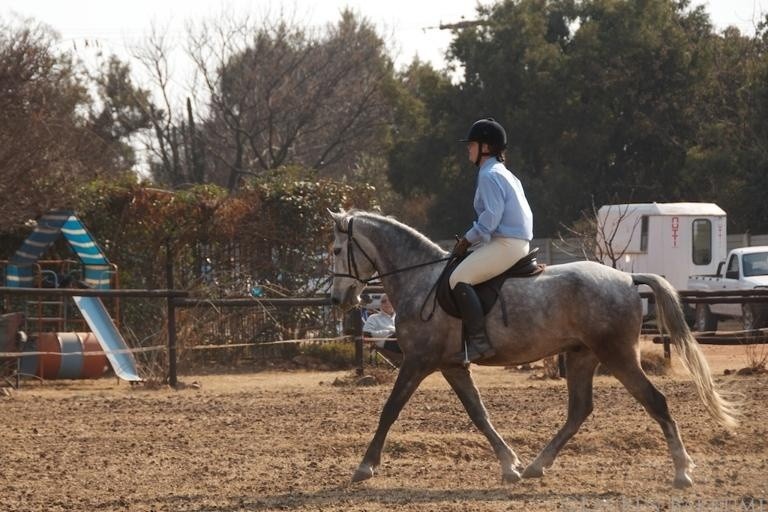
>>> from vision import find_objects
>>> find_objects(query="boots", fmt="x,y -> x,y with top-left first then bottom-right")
446,280 -> 496,366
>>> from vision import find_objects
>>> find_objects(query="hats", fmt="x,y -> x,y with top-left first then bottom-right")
457,116 -> 508,147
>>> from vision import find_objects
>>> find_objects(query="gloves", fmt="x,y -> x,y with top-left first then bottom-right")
451,236 -> 472,260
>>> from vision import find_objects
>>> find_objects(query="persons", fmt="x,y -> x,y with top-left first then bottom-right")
449,118 -> 533,364
343,295 -> 372,337
363,294 -> 402,353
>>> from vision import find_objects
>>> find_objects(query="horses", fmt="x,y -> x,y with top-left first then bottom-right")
325,206 -> 753,489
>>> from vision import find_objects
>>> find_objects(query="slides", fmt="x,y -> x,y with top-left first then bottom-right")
73,296 -> 144,383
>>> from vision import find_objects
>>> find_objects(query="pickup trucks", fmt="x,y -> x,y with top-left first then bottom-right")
687,246 -> 767,338
